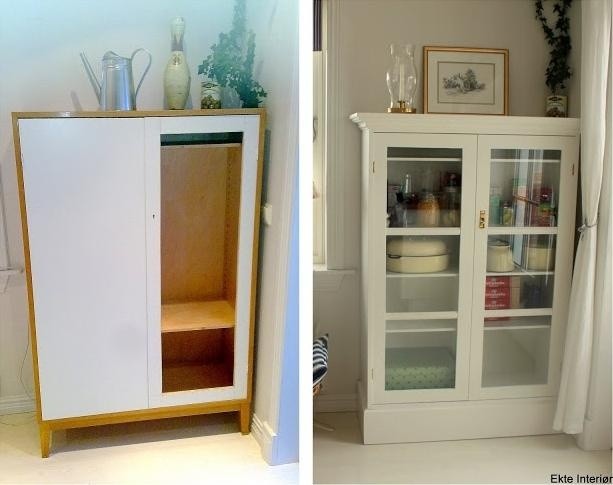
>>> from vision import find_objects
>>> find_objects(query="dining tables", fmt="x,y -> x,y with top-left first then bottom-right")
196,1 -> 268,108
533,0 -> 574,117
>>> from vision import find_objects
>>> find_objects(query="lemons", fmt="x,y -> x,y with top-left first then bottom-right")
79,47 -> 152,111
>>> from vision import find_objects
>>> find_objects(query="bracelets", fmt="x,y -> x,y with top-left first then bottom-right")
522,243 -> 555,272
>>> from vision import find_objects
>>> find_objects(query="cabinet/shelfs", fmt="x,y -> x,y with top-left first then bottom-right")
394,173 -> 415,229
502,200 -> 511,225
161,16 -> 190,110
439,172 -> 459,227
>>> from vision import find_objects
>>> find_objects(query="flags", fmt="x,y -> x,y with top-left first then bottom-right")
162,15 -> 191,110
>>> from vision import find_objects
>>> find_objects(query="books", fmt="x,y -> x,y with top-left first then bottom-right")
384,346 -> 455,390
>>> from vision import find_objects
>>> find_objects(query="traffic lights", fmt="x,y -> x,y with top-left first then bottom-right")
313,333 -> 329,390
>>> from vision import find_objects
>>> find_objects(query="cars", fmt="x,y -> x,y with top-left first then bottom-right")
488,195 -> 499,227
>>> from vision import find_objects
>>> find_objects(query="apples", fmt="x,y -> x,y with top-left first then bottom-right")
398,62 -> 406,101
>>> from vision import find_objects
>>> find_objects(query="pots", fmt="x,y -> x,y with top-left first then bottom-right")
487,237 -> 515,273
385,235 -> 449,274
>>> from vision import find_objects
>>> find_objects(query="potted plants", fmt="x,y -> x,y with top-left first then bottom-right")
422,45 -> 509,116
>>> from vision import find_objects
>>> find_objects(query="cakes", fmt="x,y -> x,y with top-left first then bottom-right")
499,199 -> 515,225
439,186 -> 460,227
546,94 -> 568,117
417,191 -> 440,227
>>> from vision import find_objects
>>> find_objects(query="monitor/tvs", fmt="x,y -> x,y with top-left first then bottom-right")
484,275 -> 523,322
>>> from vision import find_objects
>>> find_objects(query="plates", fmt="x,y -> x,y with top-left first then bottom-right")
349,111 -> 579,446
10,109 -> 267,460
